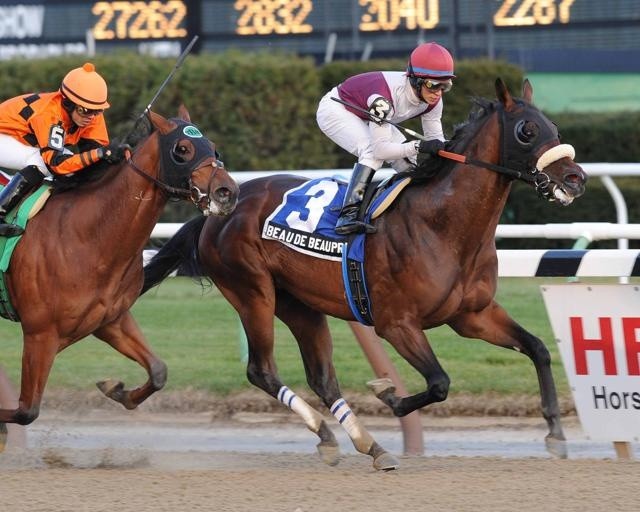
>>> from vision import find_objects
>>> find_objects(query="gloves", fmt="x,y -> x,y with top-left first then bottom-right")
97,143 -> 131,164
418,137 -> 446,158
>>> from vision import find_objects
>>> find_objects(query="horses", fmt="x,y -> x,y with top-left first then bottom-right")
141,78 -> 589,470
0,103 -> 240,430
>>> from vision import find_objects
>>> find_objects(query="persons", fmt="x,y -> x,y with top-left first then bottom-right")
0,63 -> 132,237
317,41 -> 456,235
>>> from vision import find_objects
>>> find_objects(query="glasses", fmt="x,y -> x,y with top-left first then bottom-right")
69,106 -> 103,118
411,76 -> 454,94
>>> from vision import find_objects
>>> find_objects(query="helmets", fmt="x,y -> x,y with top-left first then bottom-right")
405,42 -> 457,80
56,61 -> 111,112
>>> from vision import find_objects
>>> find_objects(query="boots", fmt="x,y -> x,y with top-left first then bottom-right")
334,163 -> 378,236
0,172 -> 32,237
